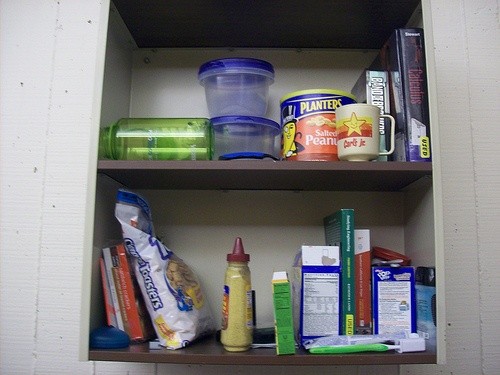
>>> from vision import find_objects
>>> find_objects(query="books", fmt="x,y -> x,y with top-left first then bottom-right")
350,27 -> 433,161
323,209 -> 371,335
100,243 -> 153,342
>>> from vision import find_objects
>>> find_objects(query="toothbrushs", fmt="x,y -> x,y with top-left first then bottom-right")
309,343 -> 401,354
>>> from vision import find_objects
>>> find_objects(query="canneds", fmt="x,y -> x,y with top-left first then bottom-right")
278,88 -> 357,161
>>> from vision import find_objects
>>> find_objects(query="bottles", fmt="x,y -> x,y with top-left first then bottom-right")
220,235 -> 253,352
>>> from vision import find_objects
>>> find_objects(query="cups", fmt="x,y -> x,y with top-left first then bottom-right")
335,103 -> 395,162
99,118 -> 214,160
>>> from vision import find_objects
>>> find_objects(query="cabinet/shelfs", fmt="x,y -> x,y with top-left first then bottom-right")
77,0 -> 446,365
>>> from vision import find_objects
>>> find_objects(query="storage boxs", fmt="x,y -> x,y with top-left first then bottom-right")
291,245 -> 343,351
371,266 -> 417,341
